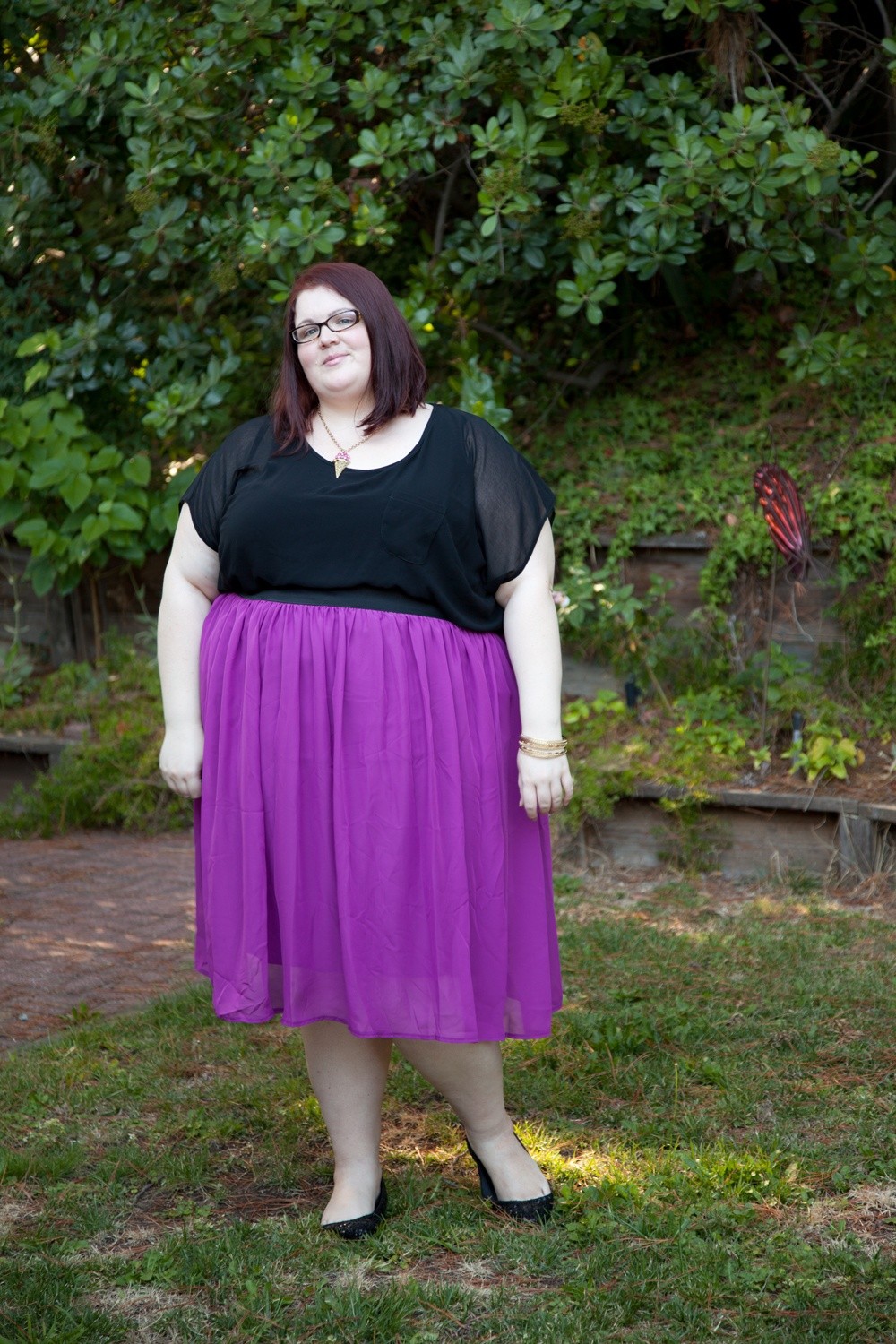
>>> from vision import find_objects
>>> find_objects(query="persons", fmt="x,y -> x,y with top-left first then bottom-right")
154,259 -> 577,1240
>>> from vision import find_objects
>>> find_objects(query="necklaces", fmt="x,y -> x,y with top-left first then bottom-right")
316,403 -> 382,479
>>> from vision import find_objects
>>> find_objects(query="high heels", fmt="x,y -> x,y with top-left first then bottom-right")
321,1182 -> 393,1240
464,1133 -> 553,1225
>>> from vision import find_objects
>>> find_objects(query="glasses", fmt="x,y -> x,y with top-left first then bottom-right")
290,309 -> 367,344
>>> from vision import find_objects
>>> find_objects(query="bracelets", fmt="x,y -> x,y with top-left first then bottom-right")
518,734 -> 568,750
518,743 -> 568,759
